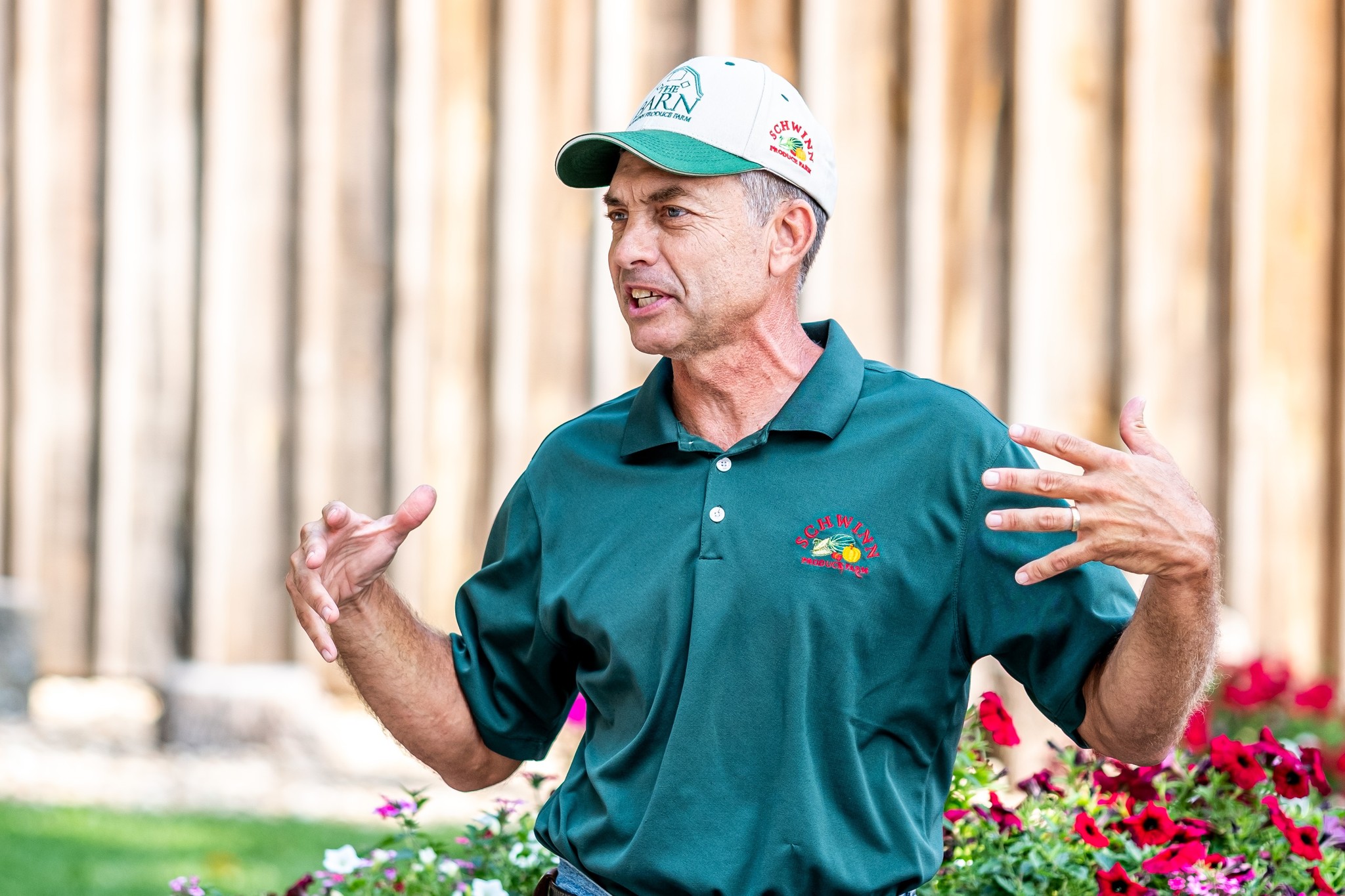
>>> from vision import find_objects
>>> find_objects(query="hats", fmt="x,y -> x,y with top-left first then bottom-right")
552,48 -> 836,223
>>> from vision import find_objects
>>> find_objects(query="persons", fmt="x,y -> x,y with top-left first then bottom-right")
284,55 -> 1219,896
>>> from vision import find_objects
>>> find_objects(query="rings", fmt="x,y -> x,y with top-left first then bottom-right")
1070,506 -> 1080,532
